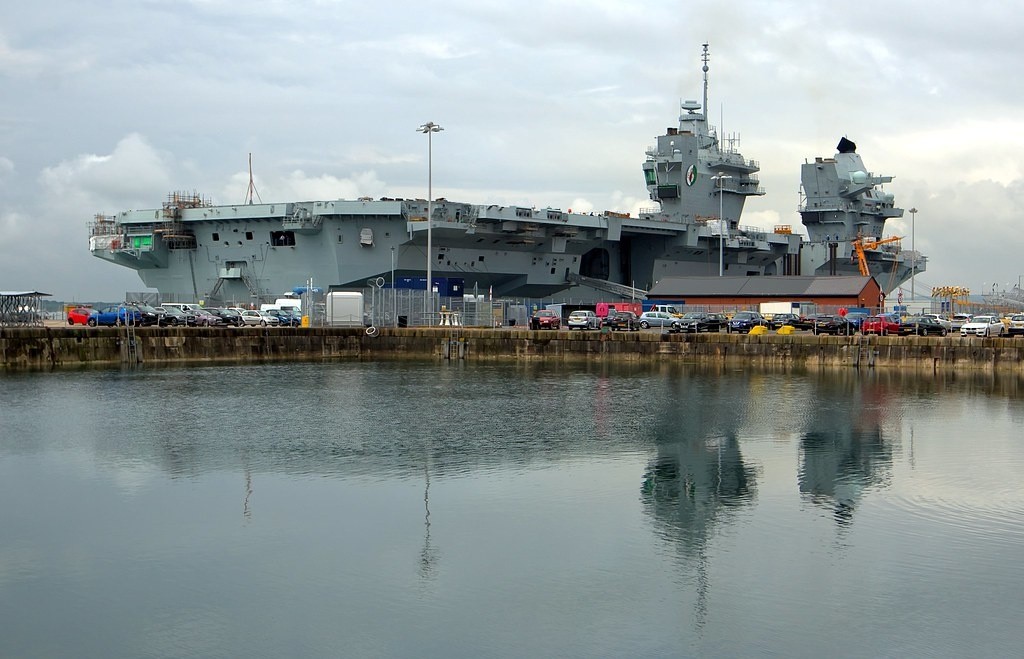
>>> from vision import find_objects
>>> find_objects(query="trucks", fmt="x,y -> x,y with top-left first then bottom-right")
596,303 -> 656,320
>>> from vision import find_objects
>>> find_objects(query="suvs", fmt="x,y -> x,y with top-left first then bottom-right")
727,312 -> 763,334
950,313 -> 974,333
656,305 -> 685,319
922,313 -> 951,333
812,315 -> 856,336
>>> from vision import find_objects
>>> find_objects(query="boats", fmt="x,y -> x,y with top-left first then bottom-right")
85,40 -> 932,317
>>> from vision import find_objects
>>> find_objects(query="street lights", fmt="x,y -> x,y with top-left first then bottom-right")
416,123 -> 445,316
907,207 -> 917,301
710,172 -> 733,278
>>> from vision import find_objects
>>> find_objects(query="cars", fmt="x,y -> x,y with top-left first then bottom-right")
529,309 -> 561,330
610,311 -> 641,331
1006,313 -> 1024,338
877,312 -> 901,324
897,316 -> 948,337
860,314 -> 900,336
66,298 -> 304,327
639,312 -> 681,329
568,310 -> 603,331
760,313 -> 829,332
674,312 -> 731,333
959,316 -> 1006,337
600,308 -> 618,326
844,312 -> 870,332
